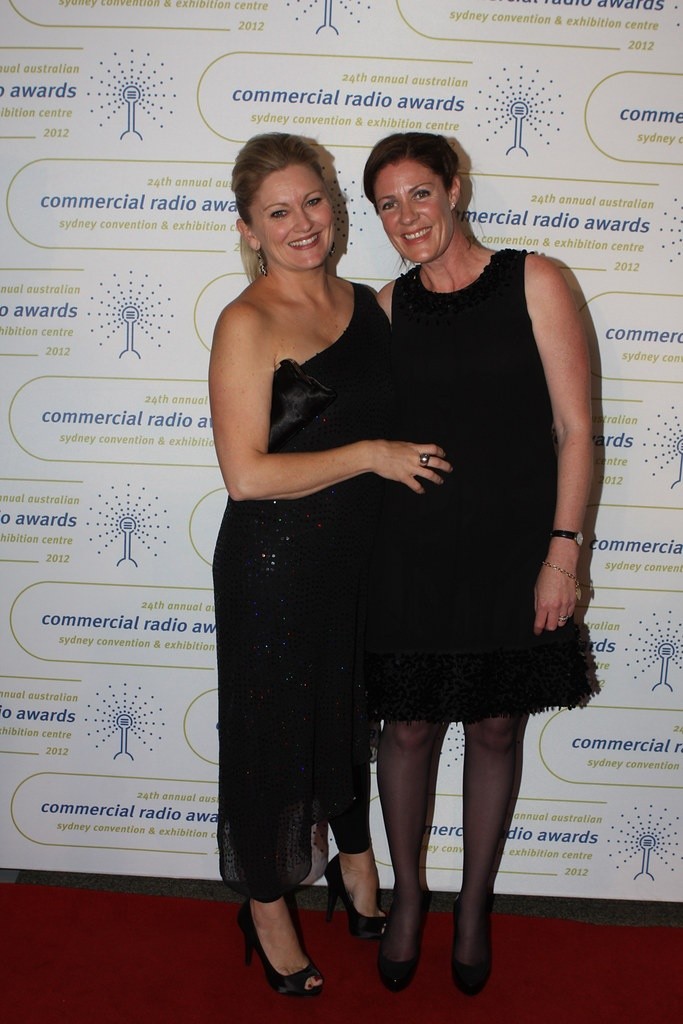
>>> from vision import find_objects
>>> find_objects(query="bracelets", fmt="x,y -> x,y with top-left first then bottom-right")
542,560 -> 587,601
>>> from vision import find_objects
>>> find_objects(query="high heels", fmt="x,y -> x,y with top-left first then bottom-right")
237,897 -> 323,996
453,893 -> 492,997
323,850 -> 388,939
377,884 -> 429,993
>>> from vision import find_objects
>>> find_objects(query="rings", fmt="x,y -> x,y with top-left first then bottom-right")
557,615 -> 568,622
419,453 -> 430,467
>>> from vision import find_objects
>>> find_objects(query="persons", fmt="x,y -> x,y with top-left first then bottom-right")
361,132 -> 598,1000
204,132 -> 457,1003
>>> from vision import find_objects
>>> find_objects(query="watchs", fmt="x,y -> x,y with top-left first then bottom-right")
549,530 -> 584,547
